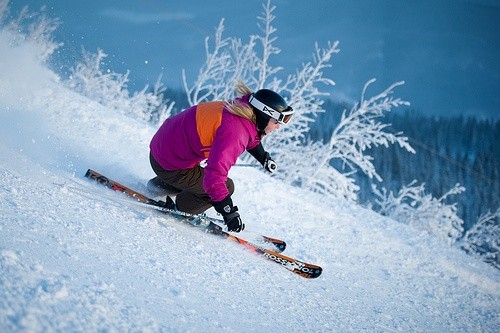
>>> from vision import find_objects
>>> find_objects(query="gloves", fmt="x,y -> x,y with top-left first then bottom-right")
247,142 -> 278,174
210,194 -> 243,233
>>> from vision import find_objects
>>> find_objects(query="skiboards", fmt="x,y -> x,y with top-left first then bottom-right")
83,167 -> 323,280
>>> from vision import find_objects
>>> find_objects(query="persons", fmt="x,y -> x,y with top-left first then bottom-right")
148,80 -> 293,233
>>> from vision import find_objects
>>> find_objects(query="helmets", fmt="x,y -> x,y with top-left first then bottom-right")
251,88 -> 295,118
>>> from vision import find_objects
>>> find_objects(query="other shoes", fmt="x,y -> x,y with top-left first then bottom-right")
172,206 -> 209,227
147,183 -> 176,198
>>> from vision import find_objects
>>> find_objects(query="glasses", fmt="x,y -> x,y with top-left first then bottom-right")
248,94 -> 294,125
